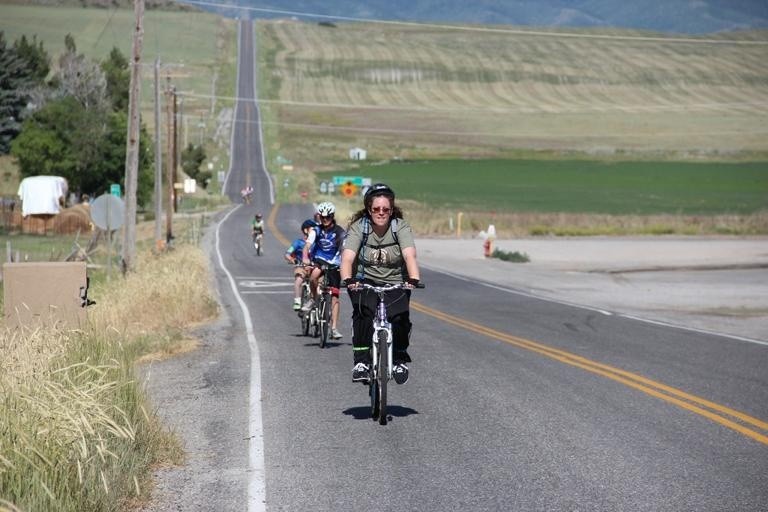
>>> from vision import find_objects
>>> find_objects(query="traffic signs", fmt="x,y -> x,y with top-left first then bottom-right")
333,176 -> 362,186
110,185 -> 121,199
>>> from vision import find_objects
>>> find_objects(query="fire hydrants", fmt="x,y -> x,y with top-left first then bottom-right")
483,240 -> 491,258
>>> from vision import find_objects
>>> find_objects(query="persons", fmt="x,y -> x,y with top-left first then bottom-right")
240,186 -> 248,203
246,186 -> 253,198
284,218 -> 323,312
313,209 -> 320,225
301,201 -> 349,341
250,212 -> 265,254
340,183 -> 421,386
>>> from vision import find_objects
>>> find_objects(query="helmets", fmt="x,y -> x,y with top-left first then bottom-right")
255,213 -> 262,219
316,201 -> 336,216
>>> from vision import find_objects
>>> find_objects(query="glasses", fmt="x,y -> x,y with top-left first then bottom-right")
319,215 -> 331,221
370,207 -> 391,214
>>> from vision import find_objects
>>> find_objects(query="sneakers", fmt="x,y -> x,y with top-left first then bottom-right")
329,328 -> 344,339
293,297 -> 317,311
393,363 -> 409,385
351,363 -> 369,381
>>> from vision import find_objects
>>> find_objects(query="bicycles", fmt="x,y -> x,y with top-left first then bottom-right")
287,258 -> 426,426
251,231 -> 263,256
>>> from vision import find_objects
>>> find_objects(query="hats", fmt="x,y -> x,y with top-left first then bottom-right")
363,183 -> 389,203
302,219 -> 316,229
